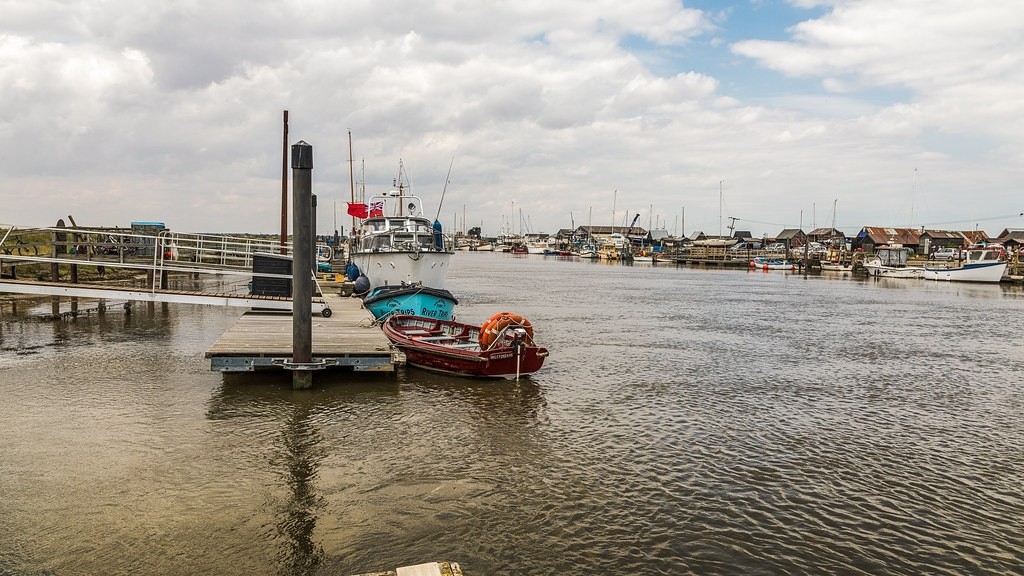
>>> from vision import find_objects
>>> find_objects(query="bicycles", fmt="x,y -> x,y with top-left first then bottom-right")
76,233 -> 137,256
0,235 -> 39,256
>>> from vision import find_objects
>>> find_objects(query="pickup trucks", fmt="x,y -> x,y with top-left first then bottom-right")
929,247 -> 966,261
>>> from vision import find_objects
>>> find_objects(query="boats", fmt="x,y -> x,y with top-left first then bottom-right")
349,156 -> 456,289
753,256 -> 806,270
862,260 -> 925,279
925,249 -> 1008,284
362,280 -> 458,324
820,260 -> 853,271
382,311 -> 550,380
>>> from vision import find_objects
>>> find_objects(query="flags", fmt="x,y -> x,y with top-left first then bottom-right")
365,202 -> 383,213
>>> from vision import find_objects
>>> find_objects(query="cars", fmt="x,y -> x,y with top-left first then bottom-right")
763,242 -> 785,250
1017,247 -> 1024,254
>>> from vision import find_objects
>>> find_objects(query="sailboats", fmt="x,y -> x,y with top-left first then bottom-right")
457,189 -> 632,258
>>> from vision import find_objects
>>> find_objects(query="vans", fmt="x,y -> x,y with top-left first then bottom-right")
731,241 -> 762,250
